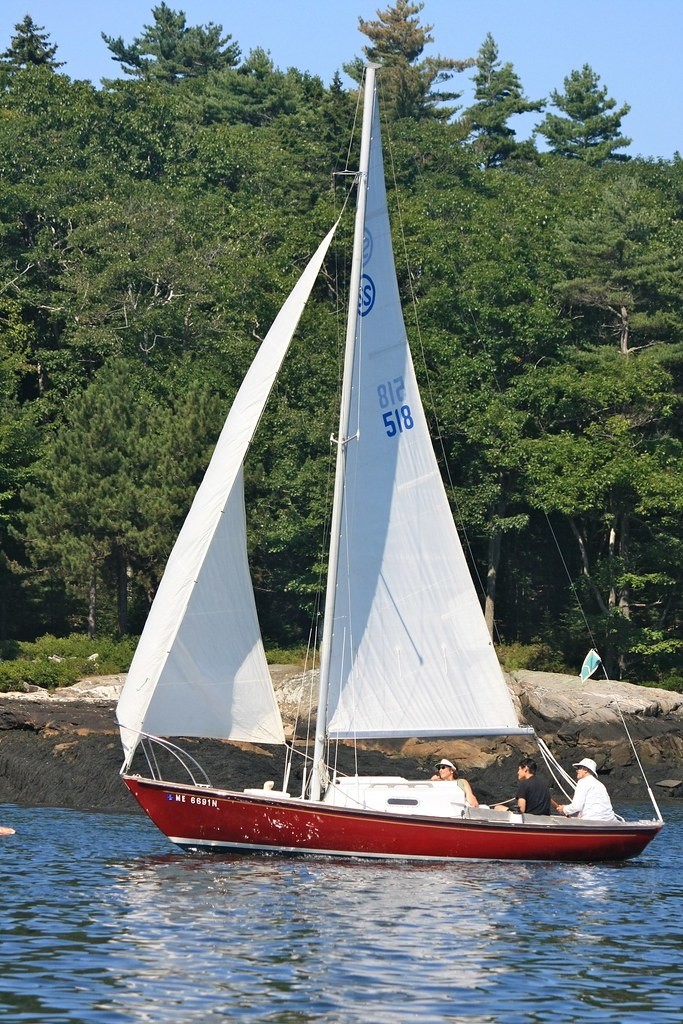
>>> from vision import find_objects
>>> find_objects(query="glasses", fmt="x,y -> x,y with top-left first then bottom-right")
438,766 -> 445,770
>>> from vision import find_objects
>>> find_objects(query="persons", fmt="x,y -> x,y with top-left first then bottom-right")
430,757 -> 490,809
494,758 -> 551,817
555,758 -> 620,823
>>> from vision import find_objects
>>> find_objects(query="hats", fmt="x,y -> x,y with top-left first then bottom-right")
572,758 -> 598,778
435,758 -> 456,771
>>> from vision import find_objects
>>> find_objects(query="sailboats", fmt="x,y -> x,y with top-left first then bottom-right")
113,66 -> 666,864
